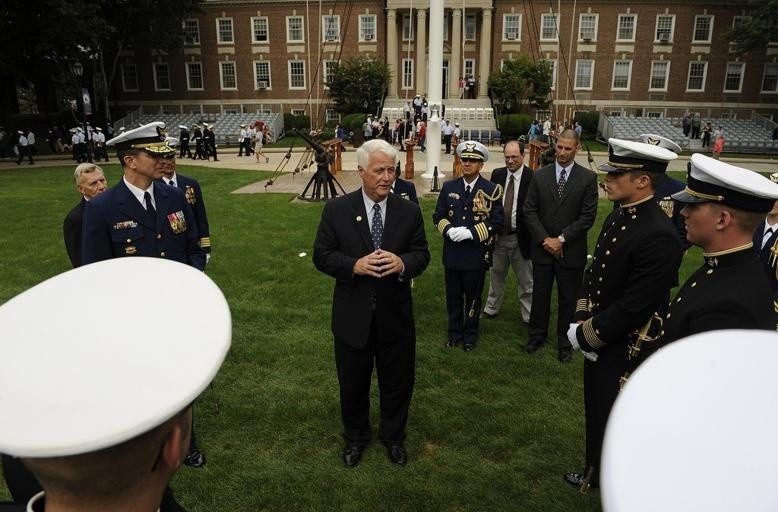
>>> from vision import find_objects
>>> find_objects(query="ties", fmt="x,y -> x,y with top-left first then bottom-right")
168,181 -> 174,187
557,169 -> 567,198
464,185 -> 471,204
503,175 -> 515,231
370,203 -> 385,249
144,190 -> 158,218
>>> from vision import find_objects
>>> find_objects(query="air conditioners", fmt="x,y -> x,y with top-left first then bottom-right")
506,31 -> 517,40
363,33 -> 373,40
660,31 -> 672,40
257,79 -> 267,89
581,31 -> 593,41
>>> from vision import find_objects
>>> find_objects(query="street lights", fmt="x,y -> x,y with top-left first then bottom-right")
70,58 -> 92,164
505,102 -> 512,142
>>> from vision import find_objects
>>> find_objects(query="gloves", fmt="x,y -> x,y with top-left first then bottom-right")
567,322 -> 598,361
446,225 -> 474,242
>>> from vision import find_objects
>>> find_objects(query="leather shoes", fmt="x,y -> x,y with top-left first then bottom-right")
342,432 -> 365,469
385,436 -> 408,466
446,338 -> 476,351
177,155 -> 222,161
521,336 -> 543,352
564,471 -> 596,487
236,150 -> 255,156
183,442 -> 206,468
558,346 -> 572,362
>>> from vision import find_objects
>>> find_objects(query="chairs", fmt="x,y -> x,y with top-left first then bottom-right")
118,112 -> 282,144
606,113 -> 777,153
387,128 -> 502,146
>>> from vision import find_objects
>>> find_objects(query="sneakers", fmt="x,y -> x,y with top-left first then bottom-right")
479,311 -> 497,321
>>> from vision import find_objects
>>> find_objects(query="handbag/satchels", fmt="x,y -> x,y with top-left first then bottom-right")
700,131 -> 706,140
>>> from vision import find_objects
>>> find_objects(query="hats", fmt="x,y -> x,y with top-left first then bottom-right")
67,126 -> 104,133
105,121 -> 176,154
671,153 -> 778,214
443,118 -> 460,126
0,256 -> 234,461
407,95 -> 428,106
178,123 -> 215,129
364,114 -> 378,125
597,133 -> 682,173
